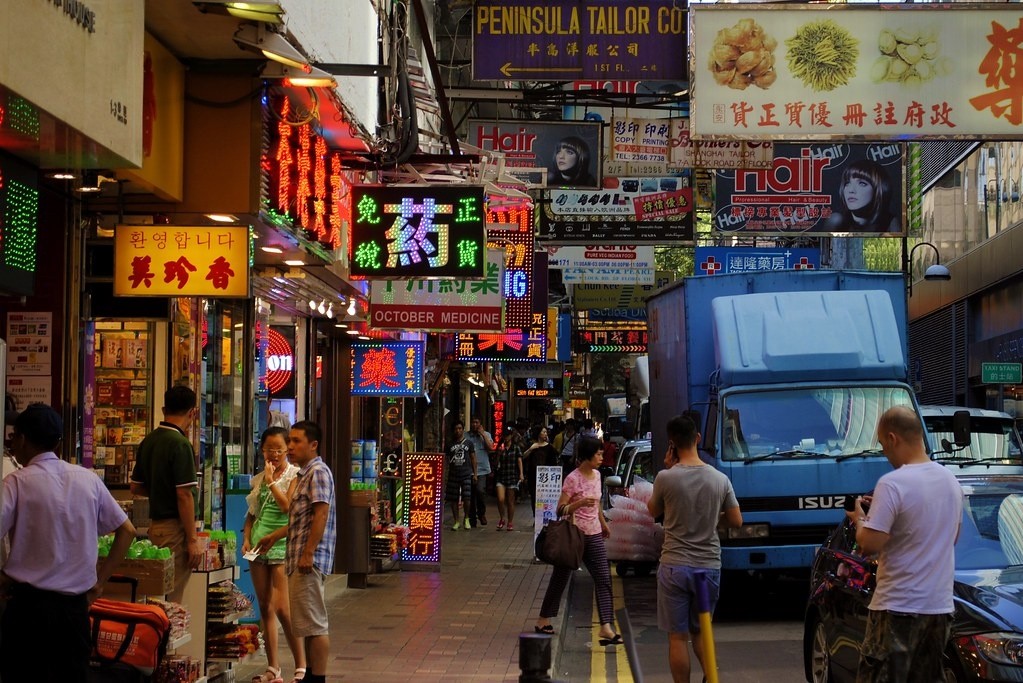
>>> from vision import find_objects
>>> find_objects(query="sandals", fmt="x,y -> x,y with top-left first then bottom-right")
289,667 -> 306,683
250,665 -> 284,683
507,522 -> 513,531
496,519 -> 505,529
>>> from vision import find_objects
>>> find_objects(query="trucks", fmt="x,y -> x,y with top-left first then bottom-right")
633,356 -> 651,439
645,270 -> 971,607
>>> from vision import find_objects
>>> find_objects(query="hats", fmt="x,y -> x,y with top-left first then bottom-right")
502,426 -> 513,435
15,402 -> 64,433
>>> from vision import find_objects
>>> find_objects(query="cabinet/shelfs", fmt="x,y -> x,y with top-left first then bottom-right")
87,315 -> 168,491
225,489 -> 267,632
165,565 -> 240,683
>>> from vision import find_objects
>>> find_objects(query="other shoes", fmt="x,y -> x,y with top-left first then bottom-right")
478,514 -> 487,525
470,520 -> 477,528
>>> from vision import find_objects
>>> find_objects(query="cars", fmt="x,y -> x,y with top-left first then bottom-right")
604,440 -> 653,496
603,414 -> 627,436
803,465 -> 1023,683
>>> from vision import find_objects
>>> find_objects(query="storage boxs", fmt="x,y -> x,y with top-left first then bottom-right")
351,489 -> 377,505
97,556 -> 176,595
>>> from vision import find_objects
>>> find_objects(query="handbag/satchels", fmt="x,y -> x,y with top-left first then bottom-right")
86,597 -> 171,677
534,504 -> 585,569
442,467 -> 450,484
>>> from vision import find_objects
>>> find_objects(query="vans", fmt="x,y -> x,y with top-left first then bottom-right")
919,405 -> 1023,466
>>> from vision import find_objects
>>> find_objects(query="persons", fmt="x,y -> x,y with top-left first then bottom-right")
285,422 -> 337,683
447,420 -> 478,532
489,412 -> 606,505
544,137 -> 599,186
534,435 -> 625,646
0,403 -> 138,683
243,426 -> 306,683
464,413 -> 494,525
819,158 -> 901,233
599,434 -> 617,482
845,405 -> 964,683
494,424 -> 524,532
524,425 -> 559,527
648,415 -> 742,683
129,385 -> 205,603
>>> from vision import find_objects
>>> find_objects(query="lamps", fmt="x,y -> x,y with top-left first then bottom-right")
202,213 -> 240,223
253,266 -> 346,317
378,128 -> 533,202
262,246 -> 283,254
192,1 -> 338,87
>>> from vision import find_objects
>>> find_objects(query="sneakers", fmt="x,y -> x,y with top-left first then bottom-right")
463,518 -> 471,529
451,522 -> 461,531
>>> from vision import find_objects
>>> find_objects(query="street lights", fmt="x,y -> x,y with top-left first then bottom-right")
903,241 -> 951,385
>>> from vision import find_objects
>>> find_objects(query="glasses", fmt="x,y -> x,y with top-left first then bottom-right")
8,432 -> 26,440
261,448 -> 289,455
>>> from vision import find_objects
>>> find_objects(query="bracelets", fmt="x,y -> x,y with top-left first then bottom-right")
267,481 -> 276,487
855,517 -> 864,523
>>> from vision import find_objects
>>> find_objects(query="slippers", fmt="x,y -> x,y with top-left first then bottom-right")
535,624 -> 555,634
598,633 -> 624,646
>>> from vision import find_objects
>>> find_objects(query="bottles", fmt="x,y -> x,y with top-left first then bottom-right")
195,529 -> 236,571
96,534 -> 171,560
349,482 -> 377,491
